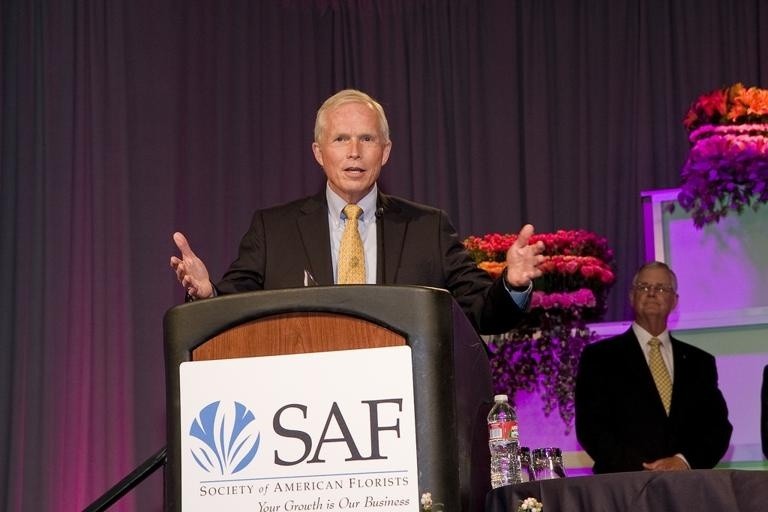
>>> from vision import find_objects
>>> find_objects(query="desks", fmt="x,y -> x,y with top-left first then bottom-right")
482,468 -> 768,511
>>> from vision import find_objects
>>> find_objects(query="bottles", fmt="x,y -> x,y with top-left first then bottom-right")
487,395 -> 521,489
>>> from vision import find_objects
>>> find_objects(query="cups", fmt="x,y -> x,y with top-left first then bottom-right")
519,446 -> 567,482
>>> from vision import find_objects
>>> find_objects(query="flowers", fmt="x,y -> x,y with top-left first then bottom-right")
458,229 -> 620,439
673,80 -> 768,230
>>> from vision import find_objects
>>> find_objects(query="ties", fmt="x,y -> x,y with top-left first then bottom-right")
648,337 -> 674,417
338,204 -> 366,285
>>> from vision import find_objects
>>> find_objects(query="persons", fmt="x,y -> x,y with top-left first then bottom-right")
760,364 -> 768,460
168,88 -> 545,334
574,261 -> 733,475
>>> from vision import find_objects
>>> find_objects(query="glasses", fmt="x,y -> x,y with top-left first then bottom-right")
635,284 -> 672,293
